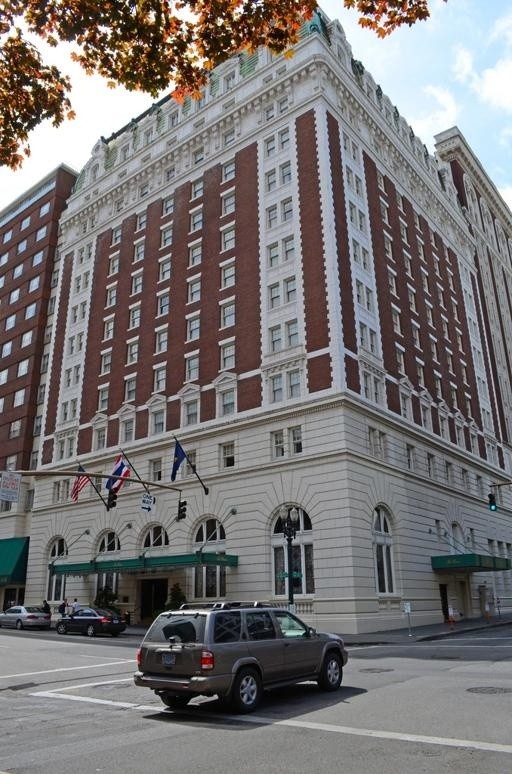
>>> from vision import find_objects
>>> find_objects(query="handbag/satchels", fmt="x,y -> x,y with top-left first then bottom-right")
64,603 -> 68,615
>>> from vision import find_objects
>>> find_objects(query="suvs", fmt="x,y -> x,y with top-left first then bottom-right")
131,599 -> 349,715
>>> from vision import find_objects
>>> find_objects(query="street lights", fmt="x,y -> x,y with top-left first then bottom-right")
279,505 -> 300,613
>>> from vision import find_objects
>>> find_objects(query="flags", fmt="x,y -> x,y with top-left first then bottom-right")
170,440 -> 186,482
68,458 -> 92,504
104,450 -> 133,495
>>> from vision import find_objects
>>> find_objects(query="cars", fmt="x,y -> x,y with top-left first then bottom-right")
1,605 -> 52,629
55,608 -> 128,636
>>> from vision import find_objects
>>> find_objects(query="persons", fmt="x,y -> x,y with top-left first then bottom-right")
39,599 -> 53,629
67,597 -> 82,611
57,598 -> 69,617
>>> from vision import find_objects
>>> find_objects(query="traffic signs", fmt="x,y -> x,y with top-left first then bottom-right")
141,493 -> 154,512
293,571 -> 302,578
276,571 -> 288,576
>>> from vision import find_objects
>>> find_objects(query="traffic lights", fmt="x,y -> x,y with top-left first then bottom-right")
487,493 -> 497,511
178,500 -> 187,519
106,488 -> 118,509
